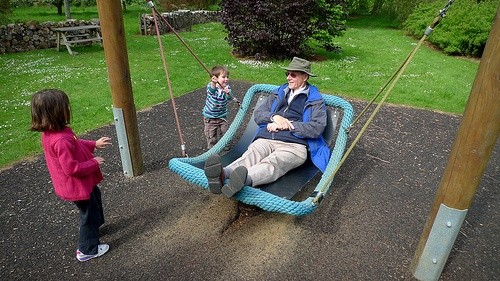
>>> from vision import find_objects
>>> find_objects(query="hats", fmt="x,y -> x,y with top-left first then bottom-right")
281,56 -> 318,77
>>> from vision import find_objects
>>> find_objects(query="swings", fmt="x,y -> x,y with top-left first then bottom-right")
147,0 -> 453,218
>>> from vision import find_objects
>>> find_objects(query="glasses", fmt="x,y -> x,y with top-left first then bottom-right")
284,72 -> 304,78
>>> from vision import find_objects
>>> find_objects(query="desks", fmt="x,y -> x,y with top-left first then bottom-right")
51,24 -> 103,56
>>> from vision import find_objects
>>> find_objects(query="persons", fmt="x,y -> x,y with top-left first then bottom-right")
201,64 -> 241,149
30,87 -> 114,262
204,56 -> 327,199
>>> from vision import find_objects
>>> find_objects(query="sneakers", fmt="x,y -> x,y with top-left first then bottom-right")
76,244 -> 110,262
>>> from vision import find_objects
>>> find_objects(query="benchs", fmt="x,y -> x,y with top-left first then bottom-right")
49,34 -> 89,46
65,37 -> 103,47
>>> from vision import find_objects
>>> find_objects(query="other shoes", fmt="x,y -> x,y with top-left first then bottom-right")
221,166 -> 248,198
204,154 -> 225,194
